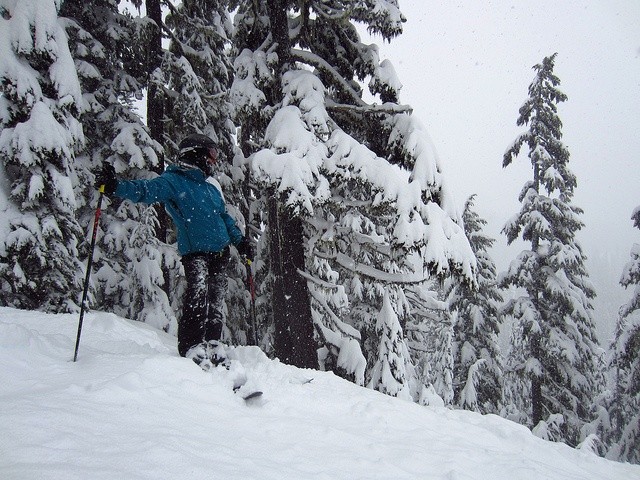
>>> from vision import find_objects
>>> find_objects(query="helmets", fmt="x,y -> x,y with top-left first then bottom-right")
176,134 -> 219,177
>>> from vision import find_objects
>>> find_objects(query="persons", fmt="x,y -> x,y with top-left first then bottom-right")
94,133 -> 255,370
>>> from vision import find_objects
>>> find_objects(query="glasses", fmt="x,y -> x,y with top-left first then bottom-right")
179,146 -> 220,161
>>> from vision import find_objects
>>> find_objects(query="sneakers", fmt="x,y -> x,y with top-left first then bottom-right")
206,340 -> 230,372
179,339 -> 221,373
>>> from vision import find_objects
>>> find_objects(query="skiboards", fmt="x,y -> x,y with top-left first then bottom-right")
204,364 -> 315,401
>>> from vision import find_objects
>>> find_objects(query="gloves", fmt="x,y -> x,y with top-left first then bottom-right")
236,241 -> 254,261
94,166 -> 119,199
